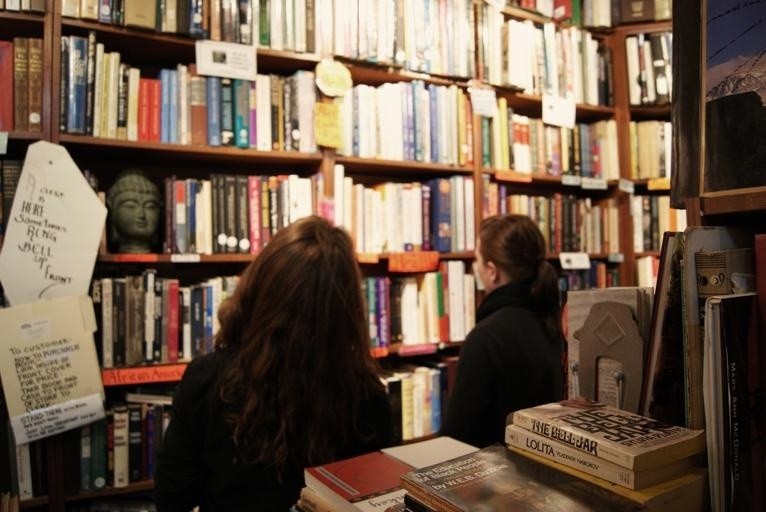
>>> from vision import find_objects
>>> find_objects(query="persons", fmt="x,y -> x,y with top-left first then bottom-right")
440,213 -> 568,451
152,214 -> 387,512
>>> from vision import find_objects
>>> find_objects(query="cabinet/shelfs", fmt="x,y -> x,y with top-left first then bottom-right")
0,1 -> 688,511
668,0 -> 766,511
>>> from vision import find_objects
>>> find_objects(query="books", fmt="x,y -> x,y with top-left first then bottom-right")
293,395 -> 705,512
91,179 -> 687,369
376,351 -> 461,439
1,0 -> 671,179
639,224 -> 764,512
2,393 -> 174,512
